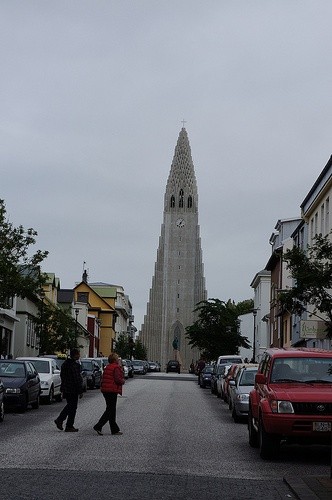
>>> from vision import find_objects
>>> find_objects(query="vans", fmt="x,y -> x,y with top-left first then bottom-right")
166,360 -> 181,374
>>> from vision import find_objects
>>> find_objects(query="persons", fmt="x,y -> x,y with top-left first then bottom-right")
93,354 -> 126,435
1,350 -> 8,360
99,352 -> 104,357
197,358 -> 206,385
54,348 -> 84,432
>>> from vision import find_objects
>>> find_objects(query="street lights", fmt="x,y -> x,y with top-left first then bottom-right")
72,307 -> 81,343
250,307 -> 261,361
37,289 -> 50,330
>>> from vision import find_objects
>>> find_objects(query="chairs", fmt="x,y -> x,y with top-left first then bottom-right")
244,373 -> 254,379
15,368 -> 24,376
275,364 -> 291,379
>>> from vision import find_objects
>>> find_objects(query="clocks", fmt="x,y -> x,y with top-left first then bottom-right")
175,218 -> 186,229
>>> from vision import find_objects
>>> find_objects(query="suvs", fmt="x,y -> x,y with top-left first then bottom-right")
247,347 -> 332,459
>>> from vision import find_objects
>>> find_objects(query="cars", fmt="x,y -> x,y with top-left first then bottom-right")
189,355 -> 259,423
149,362 -> 161,372
37,355 -> 150,397
15,356 -> 64,406
0,359 -> 42,413
0,376 -> 7,422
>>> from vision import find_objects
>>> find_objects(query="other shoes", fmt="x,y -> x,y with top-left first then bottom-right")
54,420 -> 63,430
94,425 -> 101,435
111,431 -> 123,434
65,428 -> 79,432
